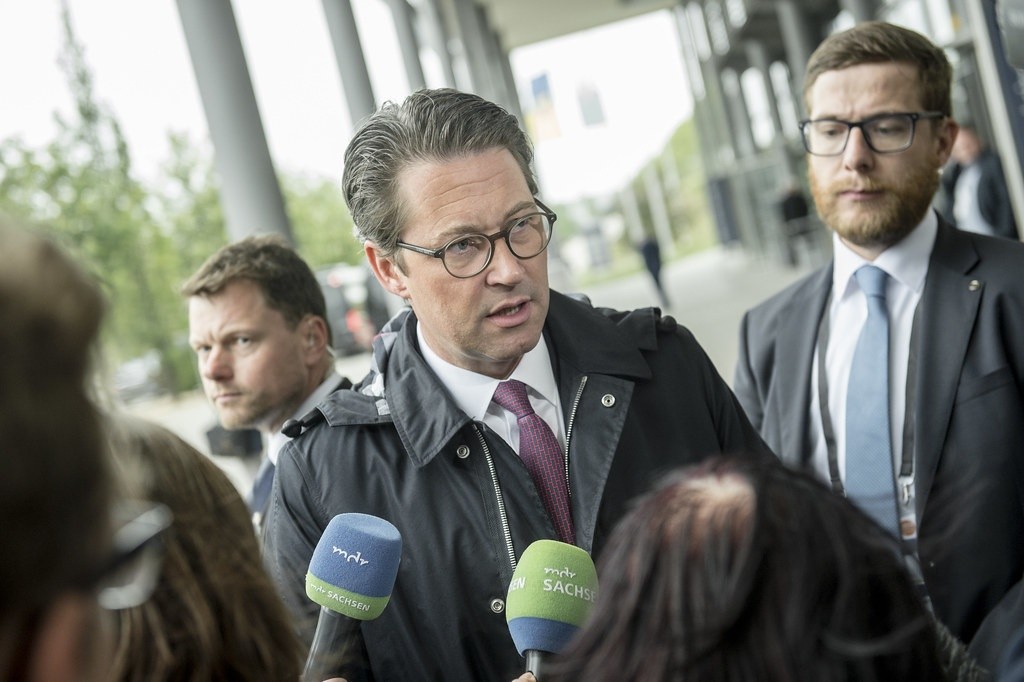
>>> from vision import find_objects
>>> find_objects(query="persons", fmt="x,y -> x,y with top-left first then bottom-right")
261,87 -> 777,682
543,461 -> 945,681
944,124 -> 1019,239
780,181 -> 814,269
0,215 -> 536,682
181,232 -> 354,536
736,22 -> 1023,682
636,236 -> 670,307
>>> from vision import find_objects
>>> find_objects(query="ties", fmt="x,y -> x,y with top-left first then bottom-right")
842,264 -> 902,545
491,379 -> 576,547
251,456 -> 277,534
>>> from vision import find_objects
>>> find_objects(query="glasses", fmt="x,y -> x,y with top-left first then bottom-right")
797,111 -> 944,157
393,198 -> 557,278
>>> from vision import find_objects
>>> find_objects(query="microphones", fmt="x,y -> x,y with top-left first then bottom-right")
506,540 -> 600,682
298,513 -> 402,682
